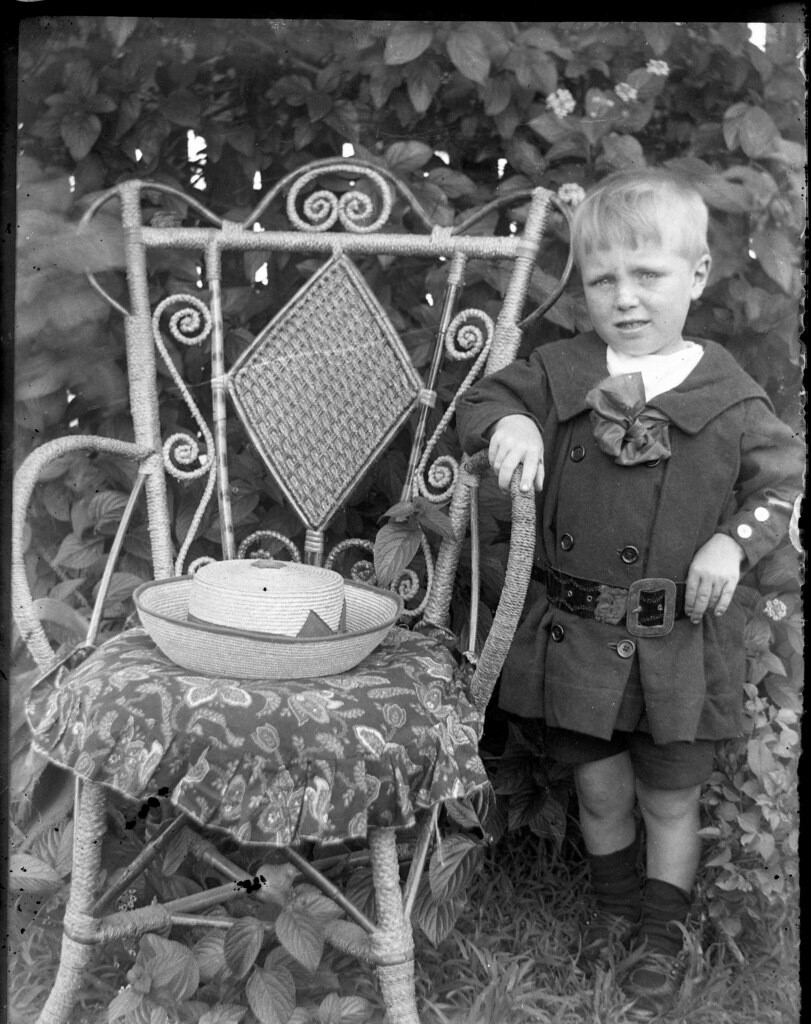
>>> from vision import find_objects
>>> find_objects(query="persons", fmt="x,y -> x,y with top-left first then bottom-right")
451,168 -> 805,1020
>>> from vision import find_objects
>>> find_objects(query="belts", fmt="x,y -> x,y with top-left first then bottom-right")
546,564 -> 704,636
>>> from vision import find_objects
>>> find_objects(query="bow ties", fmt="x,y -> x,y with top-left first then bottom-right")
584,371 -> 670,465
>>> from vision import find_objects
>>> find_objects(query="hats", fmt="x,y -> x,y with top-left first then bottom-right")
130,557 -> 405,679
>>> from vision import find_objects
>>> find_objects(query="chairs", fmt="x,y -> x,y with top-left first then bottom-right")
11,162 -> 578,1024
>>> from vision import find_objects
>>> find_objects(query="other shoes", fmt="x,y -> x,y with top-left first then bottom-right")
571,906 -> 693,1020
